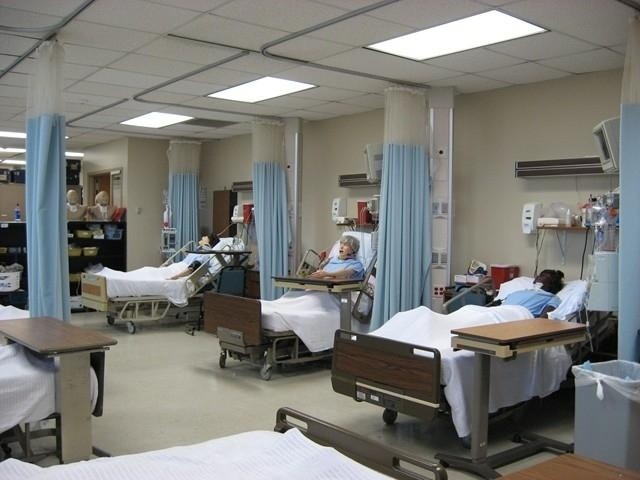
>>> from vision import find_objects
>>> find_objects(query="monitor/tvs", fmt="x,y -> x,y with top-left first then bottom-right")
363,143 -> 383,184
592,116 -> 620,174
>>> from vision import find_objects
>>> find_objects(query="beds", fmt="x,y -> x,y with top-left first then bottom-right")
326,269 -> 608,448
199,231 -> 384,381
2,306 -> 120,460
2,404 -> 449,480
81,230 -> 249,334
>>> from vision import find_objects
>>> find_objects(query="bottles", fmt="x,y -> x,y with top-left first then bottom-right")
15,203 -> 22,221
565,209 -> 572,227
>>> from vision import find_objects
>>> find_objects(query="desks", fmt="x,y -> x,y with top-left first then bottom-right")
433,318 -> 589,480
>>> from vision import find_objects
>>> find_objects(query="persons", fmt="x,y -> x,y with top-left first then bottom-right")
66,190 -> 115,220
503,270 -> 564,318
166,233 -> 220,279
312,236 -> 364,279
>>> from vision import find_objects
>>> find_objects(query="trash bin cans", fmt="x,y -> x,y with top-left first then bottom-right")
572,360 -> 640,471
